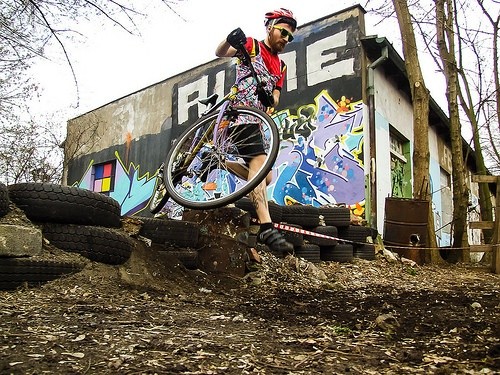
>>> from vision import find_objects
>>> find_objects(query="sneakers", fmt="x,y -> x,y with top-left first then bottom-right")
201,152 -> 218,183
257,229 -> 293,252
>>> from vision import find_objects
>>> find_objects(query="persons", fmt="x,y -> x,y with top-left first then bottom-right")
189,7 -> 300,252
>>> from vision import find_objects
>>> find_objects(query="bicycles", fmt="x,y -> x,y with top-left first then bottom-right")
150,27 -> 280,215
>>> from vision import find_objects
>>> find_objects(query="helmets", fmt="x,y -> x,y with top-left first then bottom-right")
264,8 -> 297,29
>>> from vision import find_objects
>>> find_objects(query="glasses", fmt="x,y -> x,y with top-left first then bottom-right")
273,26 -> 294,43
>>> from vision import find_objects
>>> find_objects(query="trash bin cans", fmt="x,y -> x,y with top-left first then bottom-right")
384,197 -> 430,268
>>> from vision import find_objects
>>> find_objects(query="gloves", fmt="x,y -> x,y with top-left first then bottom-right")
227,28 -> 246,49
258,90 -> 274,108
